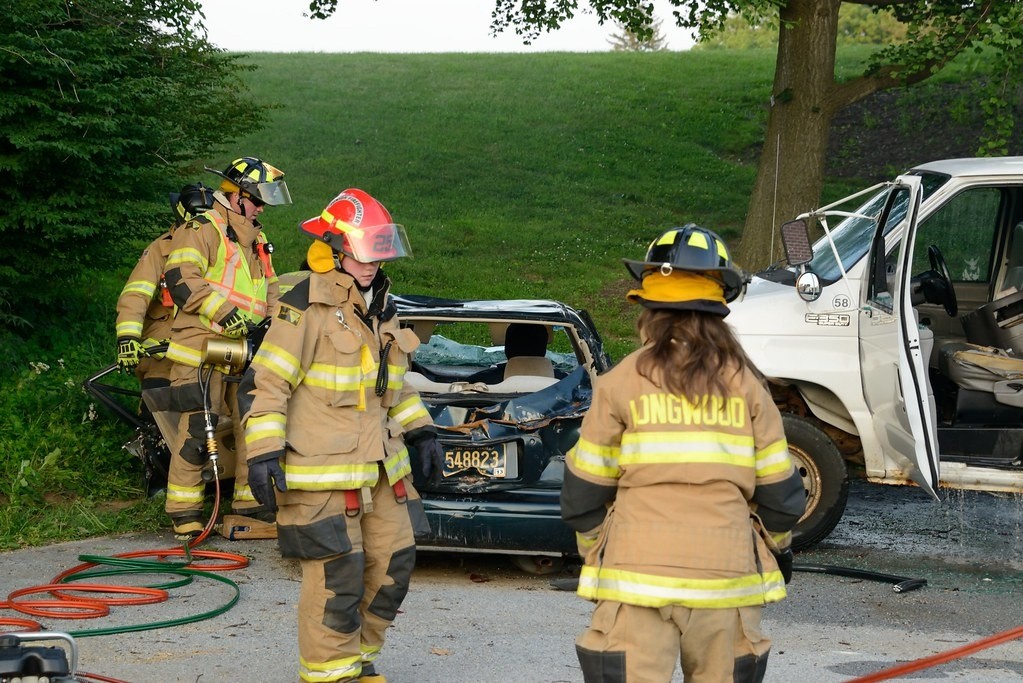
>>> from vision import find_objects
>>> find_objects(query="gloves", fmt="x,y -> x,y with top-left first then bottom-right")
116,340 -> 151,376
414,435 -> 444,492
224,311 -> 256,336
248,457 -> 287,513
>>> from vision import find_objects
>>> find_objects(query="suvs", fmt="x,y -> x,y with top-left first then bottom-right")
704,157 -> 1023,553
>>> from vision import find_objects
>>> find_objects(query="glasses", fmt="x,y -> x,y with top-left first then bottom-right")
248,195 -> 266,207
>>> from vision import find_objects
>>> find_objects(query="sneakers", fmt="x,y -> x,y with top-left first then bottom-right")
172,516 -> 204,541
358,673 -> 387,683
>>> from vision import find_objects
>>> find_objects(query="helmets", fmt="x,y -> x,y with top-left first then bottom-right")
169,182 -> 215,227
204,156 -> 292,206
299,188 -> 413,263
622,224 -> 751,319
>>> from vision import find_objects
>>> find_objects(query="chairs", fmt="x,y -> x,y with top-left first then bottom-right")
505,355 -> 557,378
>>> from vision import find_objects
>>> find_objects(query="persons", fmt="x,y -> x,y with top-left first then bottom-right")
235,189 -> 444,683
116,184 -> 218,390
463,322 -> 567,384
165,156 -> 292,540
561,225 -> 805,683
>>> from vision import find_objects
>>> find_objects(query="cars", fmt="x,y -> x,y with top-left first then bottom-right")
381,287 -> 614,579
